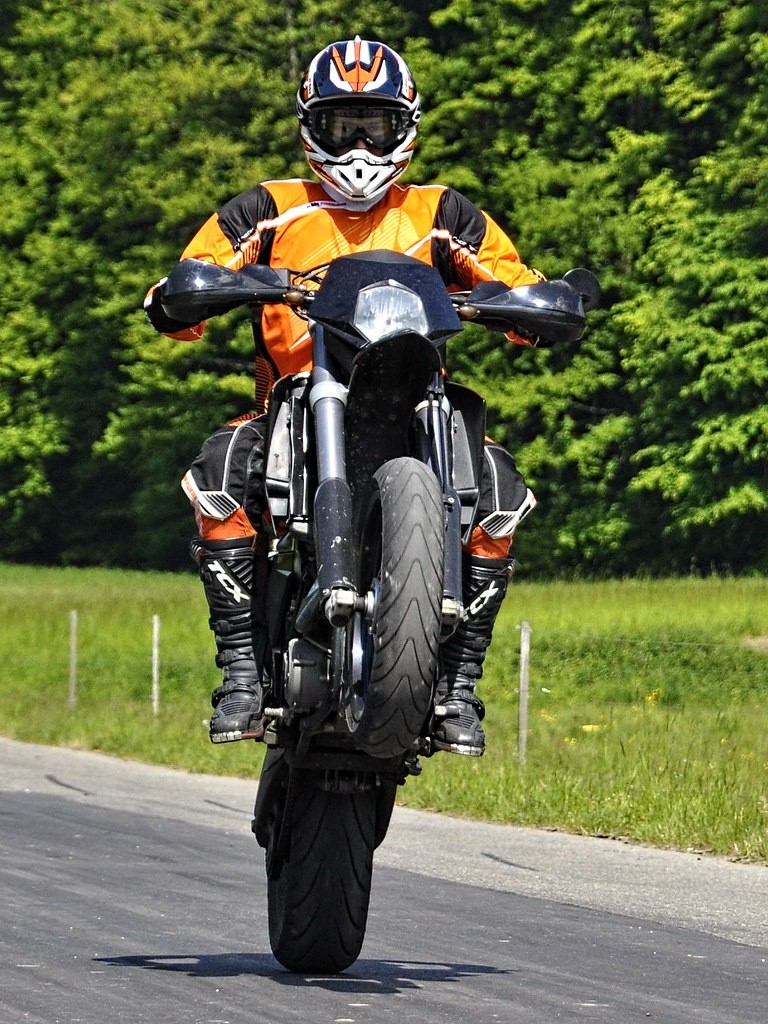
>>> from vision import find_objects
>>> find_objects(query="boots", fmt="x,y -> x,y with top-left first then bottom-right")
432,551 -> 516,758
188,534 -> 272,743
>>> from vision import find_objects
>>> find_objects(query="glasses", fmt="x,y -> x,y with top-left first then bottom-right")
297,90 -> 422,150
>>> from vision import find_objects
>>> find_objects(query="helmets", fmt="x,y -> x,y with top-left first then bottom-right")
297,35 -> 421,212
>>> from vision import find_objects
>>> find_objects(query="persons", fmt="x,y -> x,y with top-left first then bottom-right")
139,33 -> 558,758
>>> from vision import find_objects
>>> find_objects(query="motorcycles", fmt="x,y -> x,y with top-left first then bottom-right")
163,264 -> 605,980
